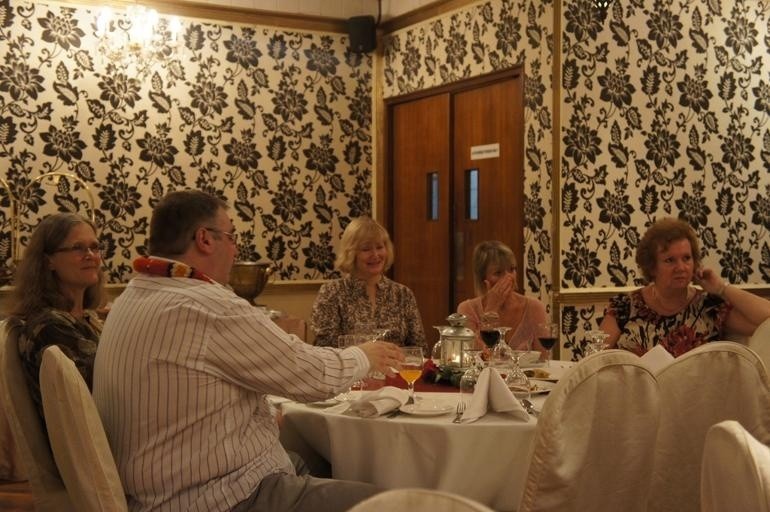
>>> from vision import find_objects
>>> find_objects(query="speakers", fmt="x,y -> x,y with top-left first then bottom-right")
348,15 -> 376,53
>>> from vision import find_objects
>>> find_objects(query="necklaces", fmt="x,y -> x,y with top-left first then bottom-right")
650,283 -> 692,312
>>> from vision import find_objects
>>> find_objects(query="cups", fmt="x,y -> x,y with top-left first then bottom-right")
337,321 -> 400,380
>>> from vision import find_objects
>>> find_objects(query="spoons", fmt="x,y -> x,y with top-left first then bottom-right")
521,400 -> 541,419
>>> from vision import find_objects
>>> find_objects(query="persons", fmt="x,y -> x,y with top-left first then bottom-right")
2,214 -> 101,433
91,189 -> 405,512
456,240 -> 549,362
311,215 -> 427,357
595,217 -> 770,355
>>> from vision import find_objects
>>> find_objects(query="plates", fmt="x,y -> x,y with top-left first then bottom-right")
517,366 -> 567,397
399,400 -> 456,416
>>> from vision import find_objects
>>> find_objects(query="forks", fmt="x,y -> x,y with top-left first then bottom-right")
386,397 -> 415,418
453,402 -> 467,424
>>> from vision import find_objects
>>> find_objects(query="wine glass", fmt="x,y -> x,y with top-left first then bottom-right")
397,346 -> 425,404
459,321 -> 530,407
537,322 -> 559,369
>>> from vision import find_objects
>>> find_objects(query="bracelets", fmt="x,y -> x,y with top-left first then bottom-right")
717,281 -> 732,299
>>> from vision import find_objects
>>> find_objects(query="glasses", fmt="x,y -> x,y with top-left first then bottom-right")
193,227 -> 238,246
58,243 -> 105,257
